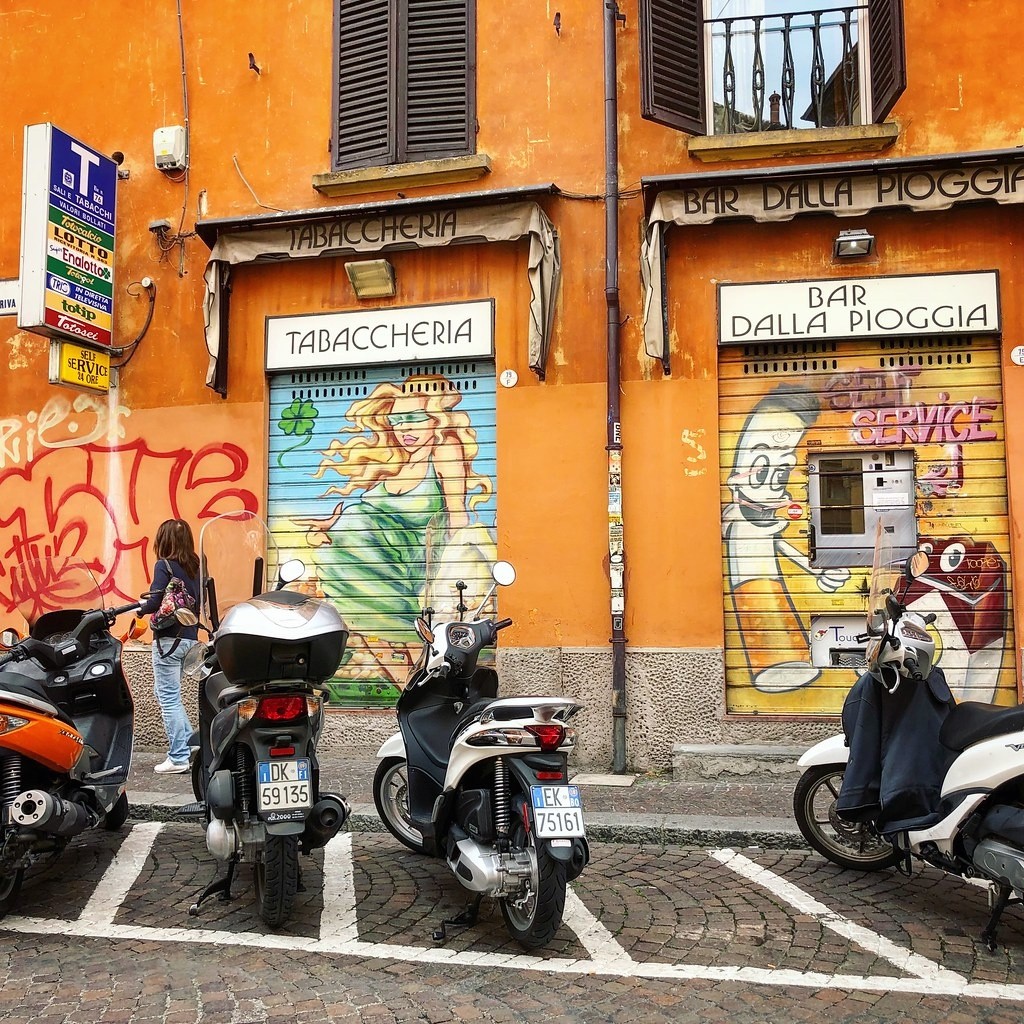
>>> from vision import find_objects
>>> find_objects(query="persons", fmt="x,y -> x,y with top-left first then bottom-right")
135,519 -> 207,774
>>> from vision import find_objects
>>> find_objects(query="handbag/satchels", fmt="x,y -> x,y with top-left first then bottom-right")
150,577 -> 196,630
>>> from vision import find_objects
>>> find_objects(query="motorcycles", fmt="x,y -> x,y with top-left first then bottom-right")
369,559 -> 590,944
172,511 -> 351,925
0,554 -> 150,919
791,551 -> 1023,952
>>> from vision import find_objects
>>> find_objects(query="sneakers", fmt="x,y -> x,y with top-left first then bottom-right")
153,746 -> 201,774
